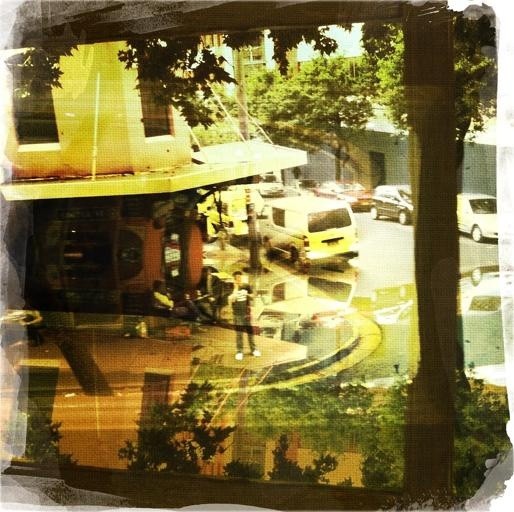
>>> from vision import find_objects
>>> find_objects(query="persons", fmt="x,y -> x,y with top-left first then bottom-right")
152,278 -> 207,333
198,267 -> 225,327
228,272 -> 262,359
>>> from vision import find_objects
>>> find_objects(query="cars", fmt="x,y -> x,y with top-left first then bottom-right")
456,191 -> 498,242
257,174 -> 285,198
311,180 -> 373,213
368,185 -> 413,224
291,178 -> 320,196
255,297 -> 357,348
373,269 -> 502,371
164,248 -> 220,285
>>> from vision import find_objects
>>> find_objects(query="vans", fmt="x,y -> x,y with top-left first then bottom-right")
258,196 -> 360,274
196,187 -> 266,247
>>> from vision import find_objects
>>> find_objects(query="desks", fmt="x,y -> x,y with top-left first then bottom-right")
174,291 -> 210,311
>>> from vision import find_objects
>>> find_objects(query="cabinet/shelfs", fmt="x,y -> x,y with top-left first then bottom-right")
122,288 -> 151,337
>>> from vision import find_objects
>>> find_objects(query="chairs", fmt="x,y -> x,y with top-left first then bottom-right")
212,283 -> 234,320
